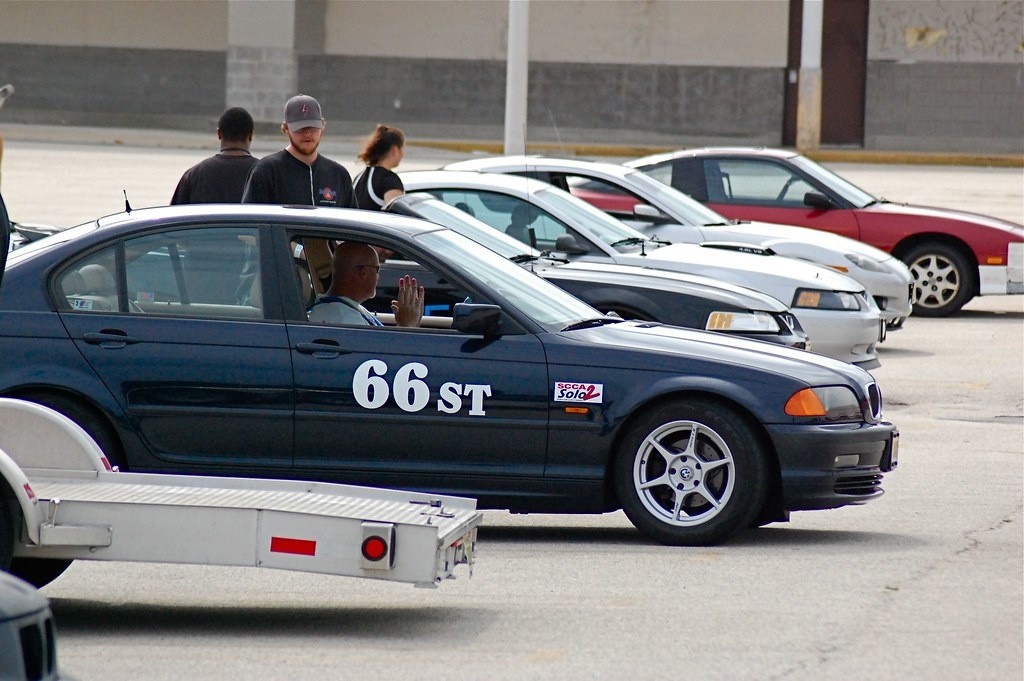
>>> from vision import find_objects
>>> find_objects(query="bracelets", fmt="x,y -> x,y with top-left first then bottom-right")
293,245 -> 304,258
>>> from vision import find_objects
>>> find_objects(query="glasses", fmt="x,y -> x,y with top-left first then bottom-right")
356,264 -> 380,273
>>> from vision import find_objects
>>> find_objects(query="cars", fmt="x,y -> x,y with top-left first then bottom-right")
0,203 -> 900,549
0,187 -> 819,356
372,169 -> 893,380
563,144 -> 1024,314
414,154 -> 919,335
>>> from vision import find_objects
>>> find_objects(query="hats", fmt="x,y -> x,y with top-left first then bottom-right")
284,93 -> 323,133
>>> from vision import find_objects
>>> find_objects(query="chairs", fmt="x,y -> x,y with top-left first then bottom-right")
79,264 -> 144,312
251,266 -> 310,311
505,203 -> 538,247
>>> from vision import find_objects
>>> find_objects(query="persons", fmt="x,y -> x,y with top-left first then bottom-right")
168,106 -> 263,304
238,94 -> 359,305
307,241 -> 426,328
351,124 -> 406,261
